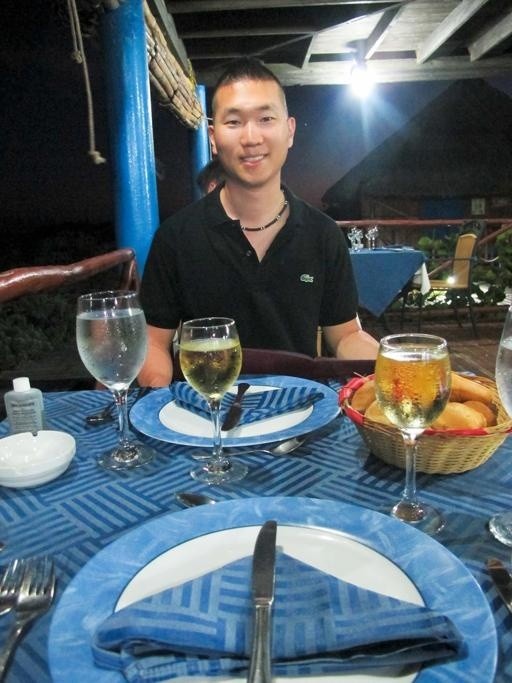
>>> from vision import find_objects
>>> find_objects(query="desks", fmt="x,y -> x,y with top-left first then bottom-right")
348,245 -> 422,341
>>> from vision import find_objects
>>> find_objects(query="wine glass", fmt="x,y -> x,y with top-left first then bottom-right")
346,223 -> 380,251
79,290 -> 160,472
373,329 -> 446,535
492,302 -> 512,548
178,317 -> 249,484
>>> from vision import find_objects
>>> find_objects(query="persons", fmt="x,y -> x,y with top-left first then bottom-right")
198,159 -> 222,197
135,61 -> 380,386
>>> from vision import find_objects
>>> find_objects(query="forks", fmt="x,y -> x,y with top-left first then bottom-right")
1,556 -> 29,618
1,556 -> 55,683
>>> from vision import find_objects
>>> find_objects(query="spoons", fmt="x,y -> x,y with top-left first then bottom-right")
189,439 -> 305,461
87,397 -> 116,424
177,491 -> 216,509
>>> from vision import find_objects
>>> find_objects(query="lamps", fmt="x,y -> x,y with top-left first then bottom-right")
346,39 -> 374,95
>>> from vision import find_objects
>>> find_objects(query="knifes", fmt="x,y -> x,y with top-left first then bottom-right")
220,383 -> 249,431
248,517 -> 284,682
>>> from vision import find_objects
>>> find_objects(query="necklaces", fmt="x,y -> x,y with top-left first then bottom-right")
226,192 -> 288,233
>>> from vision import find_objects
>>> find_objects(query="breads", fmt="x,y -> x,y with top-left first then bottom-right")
351,371 -> 497,430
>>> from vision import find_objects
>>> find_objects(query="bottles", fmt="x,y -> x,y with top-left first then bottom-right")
4,375 -> 44,437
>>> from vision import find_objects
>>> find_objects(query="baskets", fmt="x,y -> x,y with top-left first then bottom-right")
338,373 -> 512,474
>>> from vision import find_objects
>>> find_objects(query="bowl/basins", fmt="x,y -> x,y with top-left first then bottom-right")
1,430 -> 77,489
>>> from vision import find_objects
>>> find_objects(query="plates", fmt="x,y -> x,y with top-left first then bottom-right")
46,496 -> 499,683
126,370 -> 343,448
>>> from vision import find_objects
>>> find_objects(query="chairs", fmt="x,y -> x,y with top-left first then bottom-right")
400,232 -> 479,339
